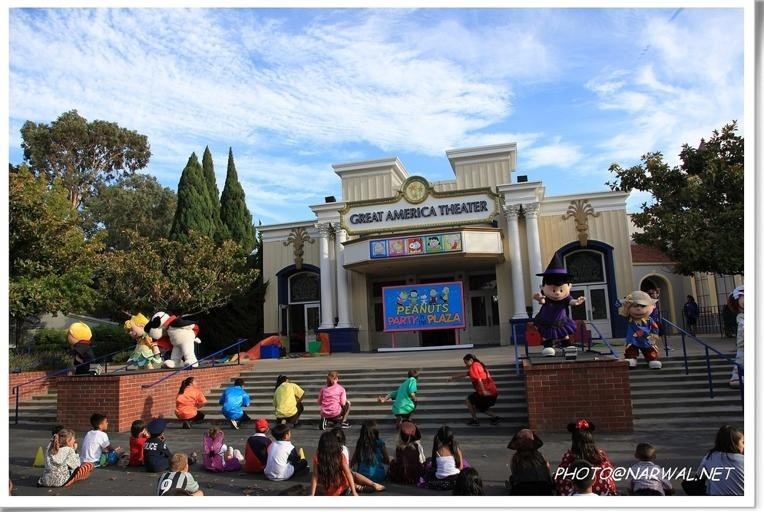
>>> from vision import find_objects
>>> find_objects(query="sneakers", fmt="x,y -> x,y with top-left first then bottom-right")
294,422 -> 301,427
395,416 -> 403,429
319,417 -> 328,431
228,446 -> 246,464
281,419 -> 287,424
183,421 -> 192,428
231,419 -> 240,430
341,421 -> 351,428
490,415 -> 504,426
466,418 -> 480,426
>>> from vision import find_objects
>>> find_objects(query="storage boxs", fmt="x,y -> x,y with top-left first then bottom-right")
260,344 -> 281,358
308,340 -> 321,353
525,330 -> 541,346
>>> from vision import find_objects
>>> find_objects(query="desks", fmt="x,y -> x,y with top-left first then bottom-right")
317,327 -> 361,353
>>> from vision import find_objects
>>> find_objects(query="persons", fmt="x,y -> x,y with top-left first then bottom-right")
317,371 -> 352,431
448,354 -> 503,425
175,376 -> 208,429
66,321 -> 104,377
684,295 -> 700,337
618,290 -> 662,368
272,375 -> 305,428
648,288 -> 674,351
218,378 -> 251,430
533,253 -> 585,359
378,369 -> 419,430
39,414 -> 744,495
727,284 -> 744,388
124,312 -> 161,371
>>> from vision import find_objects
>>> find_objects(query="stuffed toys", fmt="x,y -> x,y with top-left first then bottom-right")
144,311 -> 202,369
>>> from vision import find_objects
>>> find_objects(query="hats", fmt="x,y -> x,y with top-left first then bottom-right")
256,418 -> 269,432
147,419 -> 166,434
507,428 -> 543,451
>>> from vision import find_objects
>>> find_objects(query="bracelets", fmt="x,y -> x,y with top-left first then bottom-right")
454,376 -> 456,379
